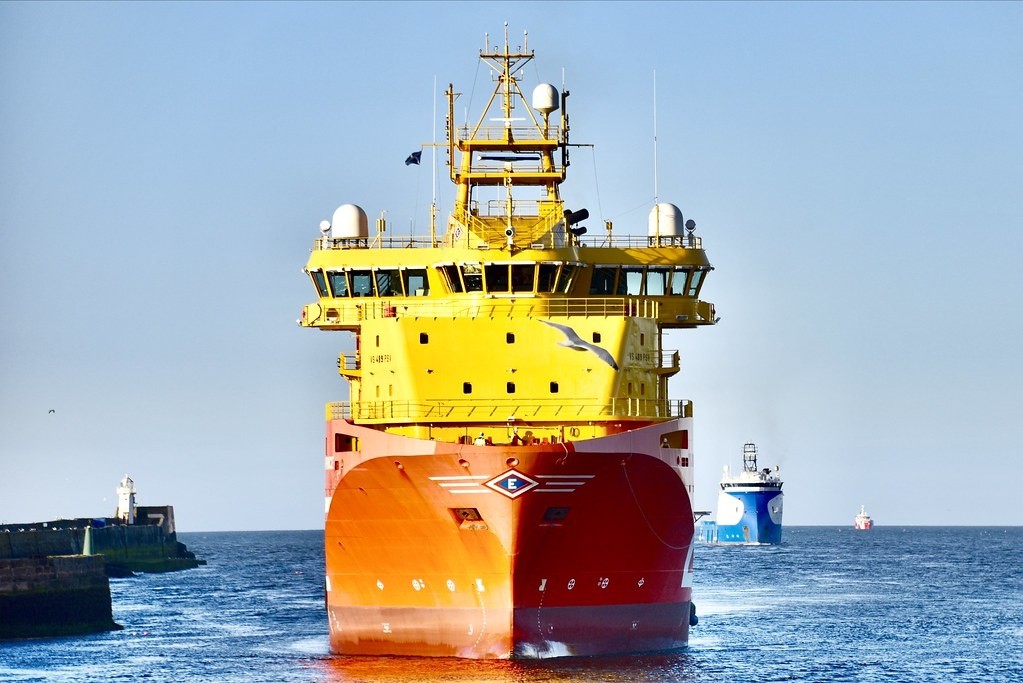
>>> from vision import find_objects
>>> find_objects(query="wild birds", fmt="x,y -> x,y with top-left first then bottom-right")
49,409 -> 55,413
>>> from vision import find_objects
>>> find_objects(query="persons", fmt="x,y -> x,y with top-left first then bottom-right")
513,433 -> 523,442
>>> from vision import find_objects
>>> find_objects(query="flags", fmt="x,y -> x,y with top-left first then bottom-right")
405,151 -> 422,165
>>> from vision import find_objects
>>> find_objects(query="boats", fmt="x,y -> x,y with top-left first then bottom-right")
699,441 -> 784,545
854,506 -> 874,529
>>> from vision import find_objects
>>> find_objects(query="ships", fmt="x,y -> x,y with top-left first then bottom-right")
297,21 -> 721,660
